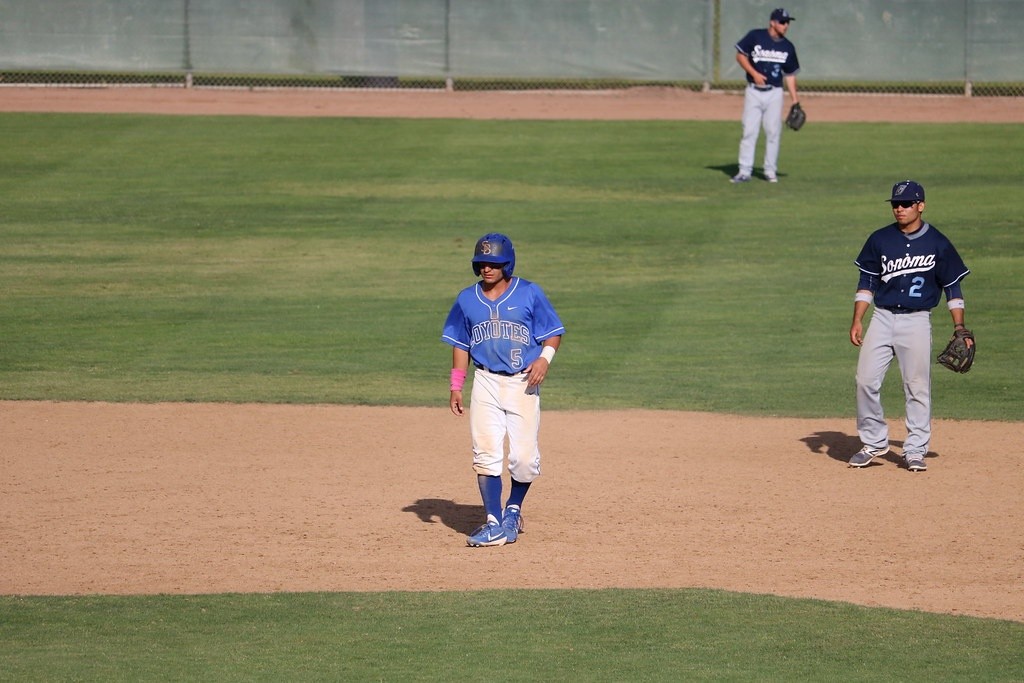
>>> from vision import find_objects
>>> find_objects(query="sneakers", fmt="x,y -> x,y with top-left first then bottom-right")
502,507 -> 523,543
465,520 -> 508,548
907,457 -> 927,472
848,443 -> 890,468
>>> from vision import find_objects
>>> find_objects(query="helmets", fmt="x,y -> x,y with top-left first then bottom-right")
470,232 -> 515,279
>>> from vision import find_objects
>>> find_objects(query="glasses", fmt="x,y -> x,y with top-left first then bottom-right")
890,200 -> 920,209
778,19 -> 790,24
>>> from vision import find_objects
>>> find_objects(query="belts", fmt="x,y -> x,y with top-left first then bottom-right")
473,362 -> 526,376
755,86 -> 772,91
876,304 -> 930,314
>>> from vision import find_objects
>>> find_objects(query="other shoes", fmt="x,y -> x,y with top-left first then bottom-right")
765,174 -> 778,183
728,172 -> 752,183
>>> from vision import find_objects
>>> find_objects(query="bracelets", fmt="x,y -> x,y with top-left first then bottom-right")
539,346 -> 556,364
855,292 -> 873,305
450,368 -> 467,391
947,300 -> 965,310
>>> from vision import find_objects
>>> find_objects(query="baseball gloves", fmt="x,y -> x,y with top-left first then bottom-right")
785,102 -> 806,131
936,327 -> 977,374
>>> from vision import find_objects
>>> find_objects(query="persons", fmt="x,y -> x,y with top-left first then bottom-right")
441,233 -> 567,548
849,180 -> 976,471
729,8 -> 806,184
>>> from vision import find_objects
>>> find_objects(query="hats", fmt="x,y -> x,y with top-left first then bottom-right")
886,180 -> 924,201
771,9 -> 795,21
